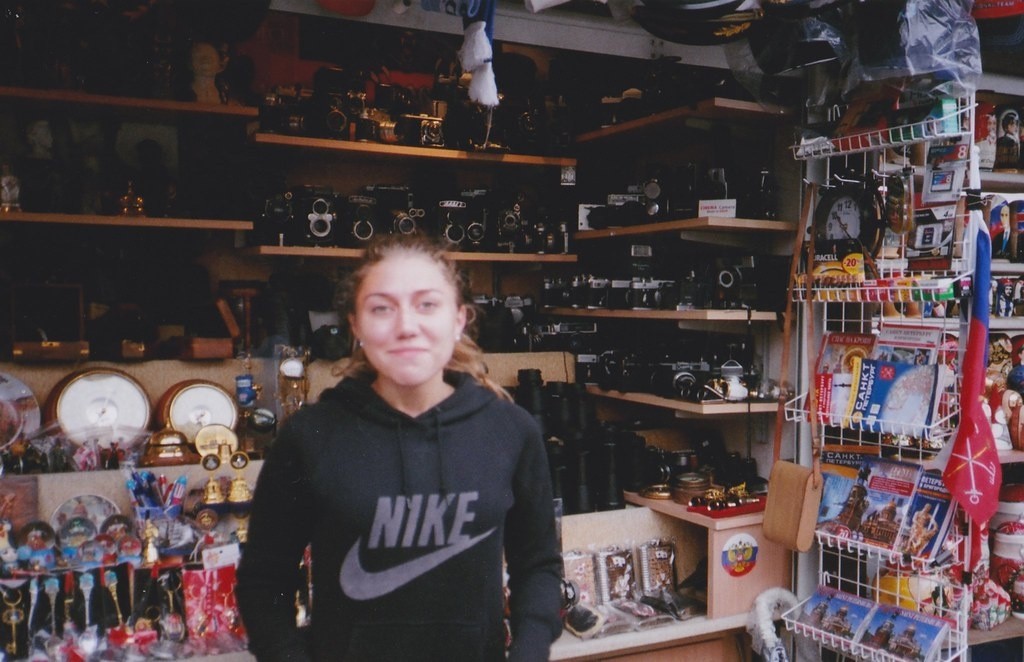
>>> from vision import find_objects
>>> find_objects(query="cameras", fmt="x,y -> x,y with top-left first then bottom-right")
262,53 -> 790,508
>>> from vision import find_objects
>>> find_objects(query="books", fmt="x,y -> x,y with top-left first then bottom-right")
814,444 -> 958,569
803,324 -> 949,438
796,583 -> 951,662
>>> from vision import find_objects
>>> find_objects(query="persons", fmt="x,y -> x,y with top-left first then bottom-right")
24,119 -> 53,158
233,229 -> 564,662
187,42 -> 222,104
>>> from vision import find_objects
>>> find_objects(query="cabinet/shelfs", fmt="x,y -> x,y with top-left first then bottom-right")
0,74 -> 1024,662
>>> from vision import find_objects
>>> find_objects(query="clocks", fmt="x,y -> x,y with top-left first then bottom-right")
811,183 -> 879,259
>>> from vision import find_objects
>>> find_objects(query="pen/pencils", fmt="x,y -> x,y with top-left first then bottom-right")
125,462 -> 188,510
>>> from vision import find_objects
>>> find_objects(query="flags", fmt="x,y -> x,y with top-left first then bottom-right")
942,214 -> 1002,526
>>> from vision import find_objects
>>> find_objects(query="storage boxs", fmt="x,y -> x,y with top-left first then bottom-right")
174,337 -> 234,360
12,341 -> 89,362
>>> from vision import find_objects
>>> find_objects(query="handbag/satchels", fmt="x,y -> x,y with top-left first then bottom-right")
762,461 -> 824,552
